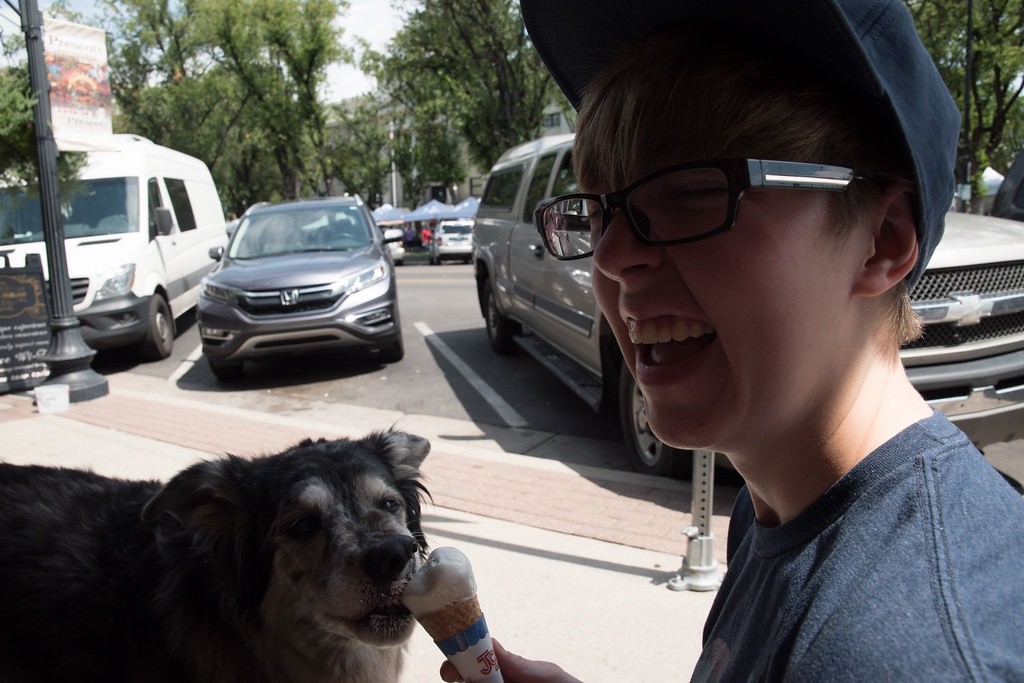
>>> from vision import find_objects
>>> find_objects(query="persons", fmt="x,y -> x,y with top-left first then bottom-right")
404,224 -> 430,252
436,0 -> 1024,683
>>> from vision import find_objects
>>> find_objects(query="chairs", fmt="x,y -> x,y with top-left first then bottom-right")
316,218 -> 362,239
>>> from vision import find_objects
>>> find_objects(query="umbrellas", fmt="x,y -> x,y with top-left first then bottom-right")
371,196 -> 482,226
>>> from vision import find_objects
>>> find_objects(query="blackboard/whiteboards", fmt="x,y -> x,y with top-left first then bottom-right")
0,254 -> 56,395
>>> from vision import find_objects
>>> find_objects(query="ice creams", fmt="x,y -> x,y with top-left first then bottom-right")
399,546 -> 483,644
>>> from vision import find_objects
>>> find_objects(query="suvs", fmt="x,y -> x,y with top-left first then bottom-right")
196,193 -> 407,380
475,133 -> 1024,485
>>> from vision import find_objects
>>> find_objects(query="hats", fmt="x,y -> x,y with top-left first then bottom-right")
521,0 -> 959,294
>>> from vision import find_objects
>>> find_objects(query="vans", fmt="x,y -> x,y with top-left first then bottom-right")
0,134 -> 225,362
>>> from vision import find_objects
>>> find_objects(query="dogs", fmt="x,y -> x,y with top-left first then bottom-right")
0,417 -> 437,683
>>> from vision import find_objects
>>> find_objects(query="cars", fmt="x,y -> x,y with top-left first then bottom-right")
428,219 -> 478,265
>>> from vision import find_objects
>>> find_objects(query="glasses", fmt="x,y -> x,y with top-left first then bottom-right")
534,159 -> 863,262
